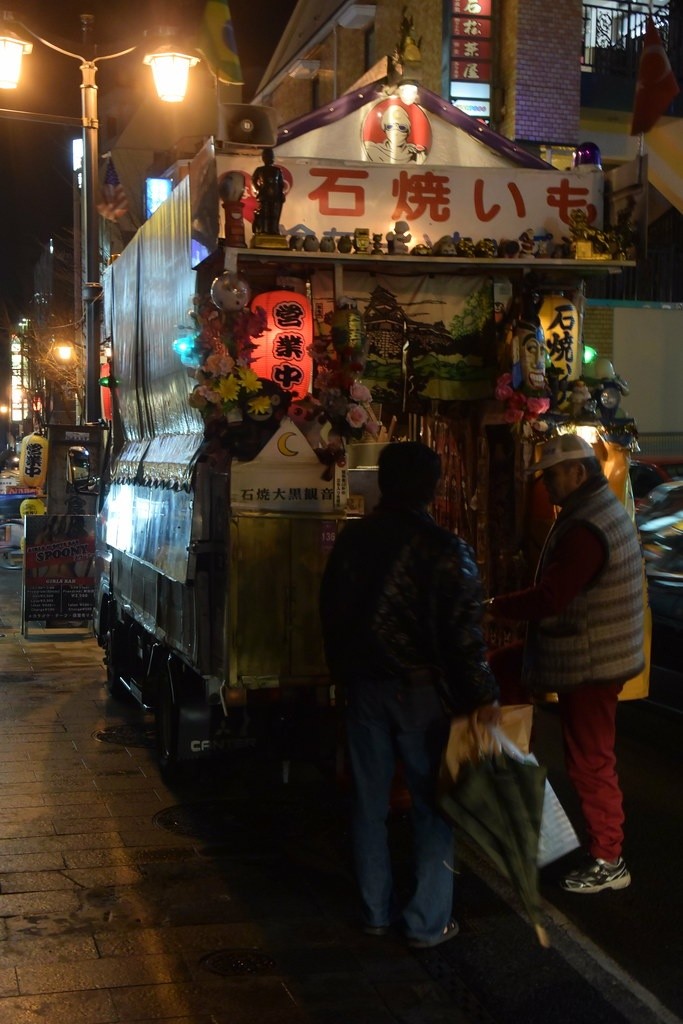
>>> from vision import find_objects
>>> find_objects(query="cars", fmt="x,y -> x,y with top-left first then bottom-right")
629,454 -> 683,589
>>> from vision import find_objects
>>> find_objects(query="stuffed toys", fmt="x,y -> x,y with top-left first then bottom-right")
222,378 -> 292,461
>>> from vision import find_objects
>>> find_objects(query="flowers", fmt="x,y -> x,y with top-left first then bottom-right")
495,372 -> 552,434
189,300 -> 377,440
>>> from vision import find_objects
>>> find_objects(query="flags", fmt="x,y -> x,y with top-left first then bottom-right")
96,158 -> 128,224
630,19 -> 680,136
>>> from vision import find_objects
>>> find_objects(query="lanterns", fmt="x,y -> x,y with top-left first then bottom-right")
249,285 -> 313,403
20,496 -> 45,521
537,291 -> 579,408
19,432 -> 48,490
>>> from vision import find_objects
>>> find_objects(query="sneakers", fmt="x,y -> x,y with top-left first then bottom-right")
561,855 -> 631,893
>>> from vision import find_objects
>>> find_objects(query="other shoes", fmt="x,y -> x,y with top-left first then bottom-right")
365,925 -> 384,936
411,917 -> 459,946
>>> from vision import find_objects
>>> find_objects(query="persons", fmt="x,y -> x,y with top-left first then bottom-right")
481,434 -> 646,893
251,148 -> 286,235
318,441 -> 503,947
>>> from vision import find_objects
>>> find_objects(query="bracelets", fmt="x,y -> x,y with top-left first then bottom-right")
486,598 -> 496,609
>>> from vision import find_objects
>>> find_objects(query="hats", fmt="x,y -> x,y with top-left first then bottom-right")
523,433 -> 595,475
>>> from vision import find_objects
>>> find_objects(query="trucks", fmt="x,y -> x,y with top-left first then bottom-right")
70,31 -> 641,796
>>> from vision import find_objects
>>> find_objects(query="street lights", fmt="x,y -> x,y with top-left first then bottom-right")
0,11 -> 200,424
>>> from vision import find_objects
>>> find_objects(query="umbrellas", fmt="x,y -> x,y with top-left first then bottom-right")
436,708 -> 548,948
